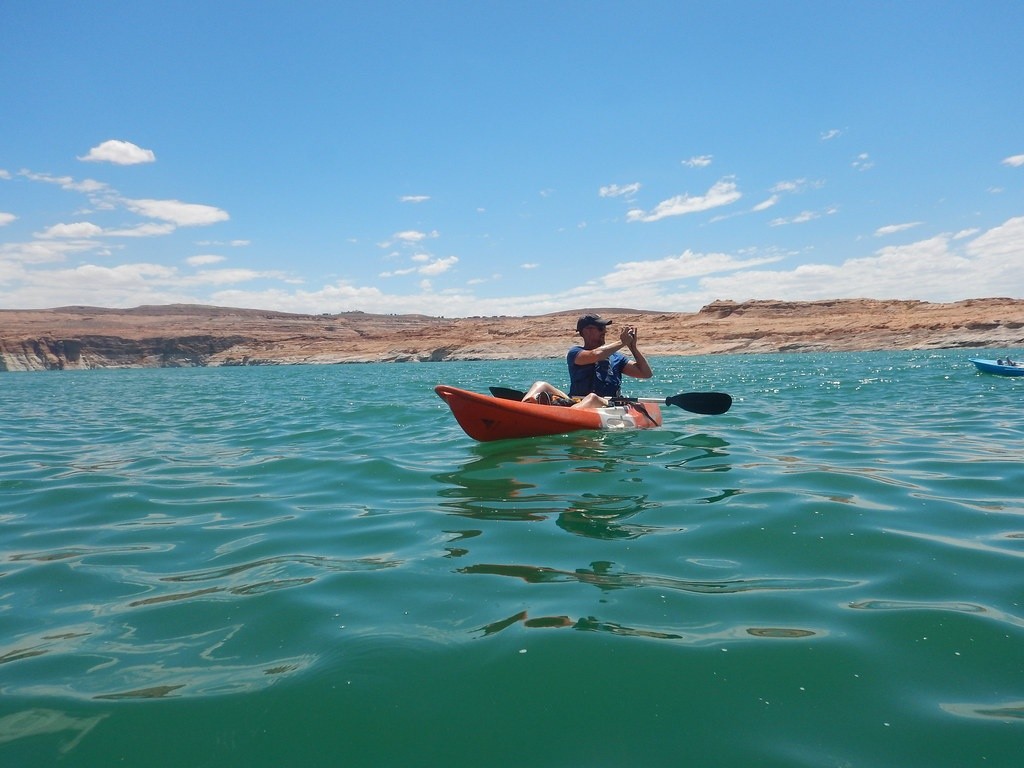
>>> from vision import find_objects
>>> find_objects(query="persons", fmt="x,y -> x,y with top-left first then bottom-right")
522,312 -> 653,407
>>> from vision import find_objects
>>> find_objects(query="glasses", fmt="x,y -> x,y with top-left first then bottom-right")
585,325 -> 606,332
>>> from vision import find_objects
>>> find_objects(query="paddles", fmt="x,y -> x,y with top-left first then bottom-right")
488,386 -> 733,416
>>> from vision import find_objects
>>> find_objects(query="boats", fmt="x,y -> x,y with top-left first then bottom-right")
433,383 -> 663,443
967,357 -> 1024,378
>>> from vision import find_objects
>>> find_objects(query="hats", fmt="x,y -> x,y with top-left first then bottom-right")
576,312 -> 612,332
